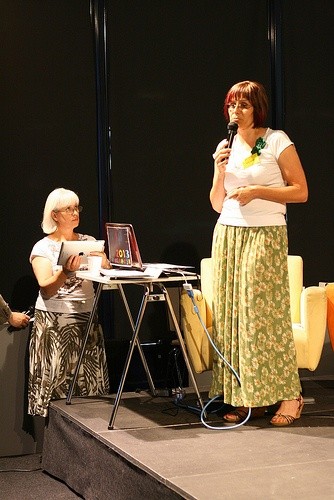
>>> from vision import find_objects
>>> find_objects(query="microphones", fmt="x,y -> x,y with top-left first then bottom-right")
222,122 -> 238,163
6,303 -> 35,335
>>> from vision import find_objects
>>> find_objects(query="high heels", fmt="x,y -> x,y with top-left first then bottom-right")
223,406 -> 252,423
269,393 -> 304,426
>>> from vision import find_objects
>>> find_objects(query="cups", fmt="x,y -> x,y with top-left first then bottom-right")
87,256 -> 101,274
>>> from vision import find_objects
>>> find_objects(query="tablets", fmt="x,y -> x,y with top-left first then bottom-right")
57,240 -> 106,265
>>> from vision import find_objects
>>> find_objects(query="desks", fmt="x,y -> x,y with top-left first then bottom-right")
66,270 -> 205,429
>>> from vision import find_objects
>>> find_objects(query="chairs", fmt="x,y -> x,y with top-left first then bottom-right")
180,254 -> 327,406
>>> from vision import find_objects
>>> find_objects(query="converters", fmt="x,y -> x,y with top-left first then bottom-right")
188,406 -> 206,415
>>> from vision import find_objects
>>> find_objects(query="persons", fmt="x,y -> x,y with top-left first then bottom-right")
209,81 -> 308,425
0,295 -> 30,327
28,188 -> 110,417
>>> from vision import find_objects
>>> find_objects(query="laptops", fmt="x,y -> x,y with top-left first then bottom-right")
104,222 -> 195,272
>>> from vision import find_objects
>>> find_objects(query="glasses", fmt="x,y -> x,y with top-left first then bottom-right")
52,205 -> 83,216
230,102 -> 253,109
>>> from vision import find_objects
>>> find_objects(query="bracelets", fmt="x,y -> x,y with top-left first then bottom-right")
63,267 -> 73,277
